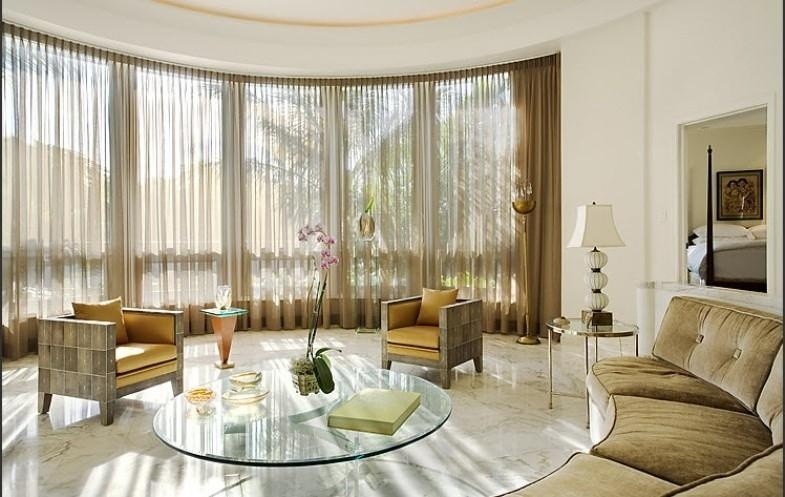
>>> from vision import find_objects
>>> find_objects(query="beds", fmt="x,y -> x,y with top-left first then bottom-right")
686,228 -> 767,283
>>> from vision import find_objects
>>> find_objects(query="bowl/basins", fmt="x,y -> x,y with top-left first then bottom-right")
191,399 -> 218,417
182,389 -> 217,405
228,371 -> 263,387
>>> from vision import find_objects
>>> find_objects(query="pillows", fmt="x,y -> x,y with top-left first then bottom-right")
693,225 -> 755,239
748,225 -> 767,239
72,297 -> 131,345
416,288 -> 459,327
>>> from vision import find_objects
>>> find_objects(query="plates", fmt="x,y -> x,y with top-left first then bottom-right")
222,387 -> 271,407
224,404 -> 272,422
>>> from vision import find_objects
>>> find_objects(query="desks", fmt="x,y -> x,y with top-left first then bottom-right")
544,319 -> 639,429
200,307 -> 248,369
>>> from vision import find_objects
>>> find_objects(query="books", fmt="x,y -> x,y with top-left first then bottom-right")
326,387 -> 421,437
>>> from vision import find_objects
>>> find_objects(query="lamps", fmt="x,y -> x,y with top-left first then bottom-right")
567,203 -> 626,326
512,199 -> 540,345
357,198 -> 379,334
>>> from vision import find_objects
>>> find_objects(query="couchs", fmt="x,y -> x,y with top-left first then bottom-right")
493,296 -> 784,497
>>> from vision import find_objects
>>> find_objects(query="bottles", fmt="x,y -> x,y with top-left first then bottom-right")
214,286 -> 233,311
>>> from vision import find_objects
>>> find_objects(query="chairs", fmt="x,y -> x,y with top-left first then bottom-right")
38,308 -> 184,427
381,296 -> 483,390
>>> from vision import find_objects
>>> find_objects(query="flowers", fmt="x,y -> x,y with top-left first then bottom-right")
291,224 -> 342,395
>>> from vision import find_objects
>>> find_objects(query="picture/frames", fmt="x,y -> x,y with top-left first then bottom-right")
717,170 -> 763,221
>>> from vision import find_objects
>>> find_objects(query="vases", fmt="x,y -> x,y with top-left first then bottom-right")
216,286 -> 232,310
288,368 -> 320,396
